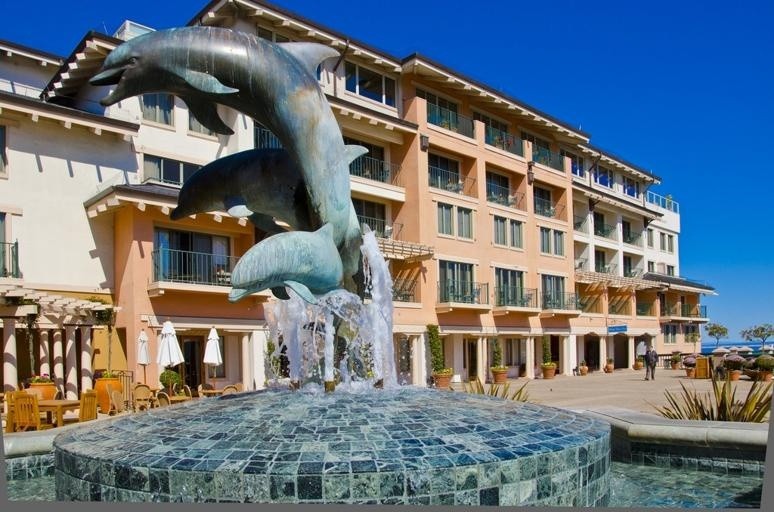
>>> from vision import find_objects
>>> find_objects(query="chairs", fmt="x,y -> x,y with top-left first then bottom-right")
5,390 -> 97,433
134,382 -> 238,409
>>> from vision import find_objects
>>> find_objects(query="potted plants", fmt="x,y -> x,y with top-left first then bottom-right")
635,356 -> 644,369
671,353 -> 774,382
432,368 -> 453,389
606,358 -> 614,372
579,360 -> 589,376
540,362 -> 557,378
94,372 -> 124,413
28,375 -> 58,400
490,366 -> 508,384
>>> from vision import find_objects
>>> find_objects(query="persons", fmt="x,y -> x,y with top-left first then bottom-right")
642,345 -> 658,381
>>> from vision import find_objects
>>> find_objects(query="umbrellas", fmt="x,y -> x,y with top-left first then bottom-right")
135,328 -> 151,383
202,325 -> 224,396
156,319 -> 186,396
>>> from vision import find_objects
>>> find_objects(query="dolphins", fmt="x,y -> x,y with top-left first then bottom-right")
228,220 -> 344,303
90,26 -> 367,249
168,144 -> 368,298
